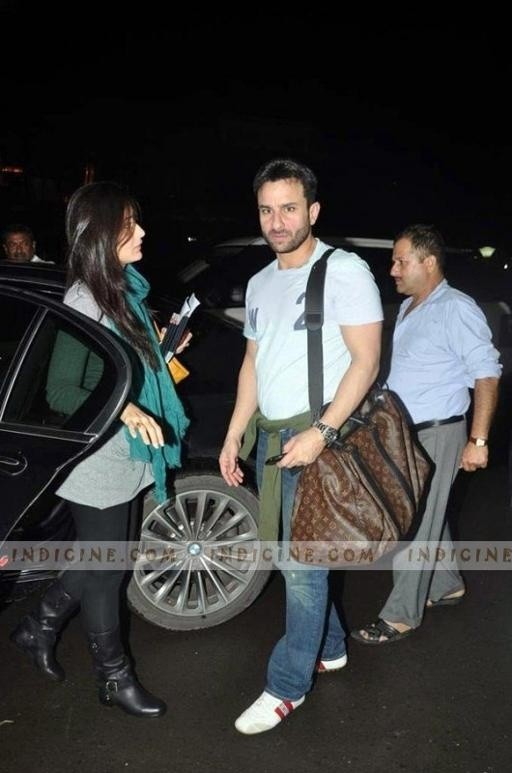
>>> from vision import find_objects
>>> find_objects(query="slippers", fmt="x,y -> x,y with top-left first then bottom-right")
352,618 -> 416,645
425,596 -> 463,609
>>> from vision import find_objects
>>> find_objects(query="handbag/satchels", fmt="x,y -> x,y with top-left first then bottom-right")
291,392 -> 430,568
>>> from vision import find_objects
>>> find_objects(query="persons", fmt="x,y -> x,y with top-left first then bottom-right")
0,226 -> 54,264
6,177 -> 199,721
356,225 -> 503,646
217,158 -> 381,739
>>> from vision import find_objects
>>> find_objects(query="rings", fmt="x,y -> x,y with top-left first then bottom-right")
136,423 -> 142,429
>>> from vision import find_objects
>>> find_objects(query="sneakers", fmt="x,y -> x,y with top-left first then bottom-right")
234,692 -> 305,735
316,654 -> 348,674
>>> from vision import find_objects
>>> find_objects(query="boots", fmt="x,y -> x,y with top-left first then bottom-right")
9,580 -> 79,681
87,626 -> 166,718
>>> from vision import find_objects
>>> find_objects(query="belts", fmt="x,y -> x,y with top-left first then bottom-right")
414,415 -> 466,432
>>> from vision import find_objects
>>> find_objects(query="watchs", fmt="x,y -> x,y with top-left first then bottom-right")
311,418 -> 340,446
468,437 -> 491,447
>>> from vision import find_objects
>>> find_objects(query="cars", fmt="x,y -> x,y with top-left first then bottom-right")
149,235 -> 489,376
0,263 -> 282,630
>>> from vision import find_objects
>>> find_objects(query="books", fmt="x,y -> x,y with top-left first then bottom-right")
157,289 -> 198,367
